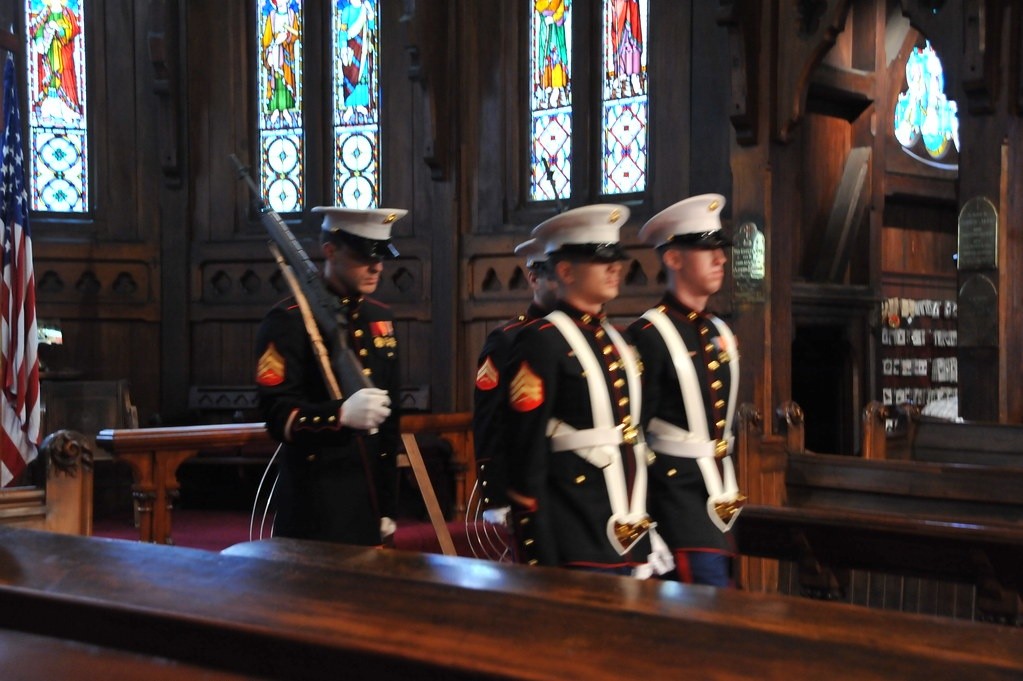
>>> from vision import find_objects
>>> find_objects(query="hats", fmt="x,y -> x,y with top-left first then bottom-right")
311,206 -> 409,263
514,238 -> 550,268
637,193 -> 733,249
530,204 -> 631,262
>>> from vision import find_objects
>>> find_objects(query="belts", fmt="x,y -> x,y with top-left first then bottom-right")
544,418 -> 641,467
646,417 -> 733,460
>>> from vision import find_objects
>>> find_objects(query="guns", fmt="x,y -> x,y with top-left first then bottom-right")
230,153 -> 379,435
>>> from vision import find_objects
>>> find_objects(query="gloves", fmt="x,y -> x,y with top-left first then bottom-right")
379,517 -> 397,542
629,515 -> 677,582
337,387 -> 392,429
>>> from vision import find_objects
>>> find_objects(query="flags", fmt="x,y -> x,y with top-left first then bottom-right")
0,52 -> 43,489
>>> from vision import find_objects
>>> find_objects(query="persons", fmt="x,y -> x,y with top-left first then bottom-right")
474,192 -> 750,587
255,205 -> 409,546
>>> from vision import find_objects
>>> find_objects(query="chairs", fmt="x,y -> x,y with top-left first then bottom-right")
41,379 -> 144,528
